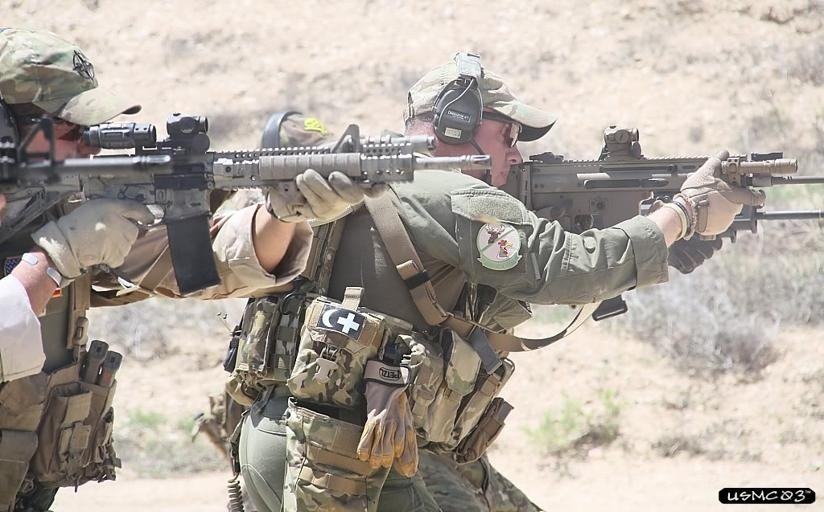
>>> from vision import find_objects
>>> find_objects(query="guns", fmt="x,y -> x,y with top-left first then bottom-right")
499,125 -> 823,321
1,113 -> 491,298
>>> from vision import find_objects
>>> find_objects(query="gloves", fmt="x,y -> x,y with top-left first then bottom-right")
667,236 -> 722,274
673,149 -> 765,236
357,361 -> 418,478
269,169 -> 389,224
31,198 -> 154,279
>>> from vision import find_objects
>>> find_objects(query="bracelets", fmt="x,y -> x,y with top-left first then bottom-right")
654,195 -> 691,240
21,253 -> 62,286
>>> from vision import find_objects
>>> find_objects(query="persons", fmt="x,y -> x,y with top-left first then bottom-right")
195,110 -> 339,453
238,51 -> 765,512
0,25 -> 388,511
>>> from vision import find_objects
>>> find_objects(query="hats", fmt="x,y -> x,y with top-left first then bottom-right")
1,27 -> 142,126
280,114 -> 339,147
403,63 -> 556,141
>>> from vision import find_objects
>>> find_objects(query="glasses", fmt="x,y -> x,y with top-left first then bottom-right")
477,113 -> 520,148
